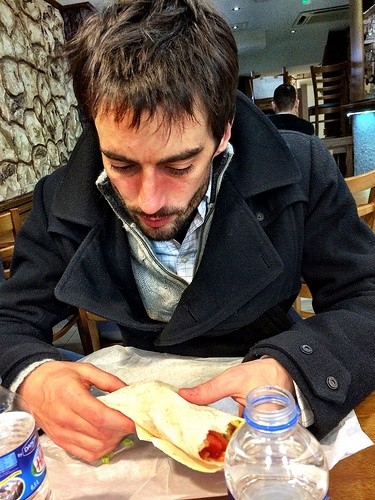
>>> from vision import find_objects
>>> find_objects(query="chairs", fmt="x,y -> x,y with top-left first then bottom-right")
0,202 -> 100,354
294,170 -> 375,319
308,61 -> 348,136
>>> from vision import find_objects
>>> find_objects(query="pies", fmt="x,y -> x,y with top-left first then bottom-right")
95,379 -> 245,473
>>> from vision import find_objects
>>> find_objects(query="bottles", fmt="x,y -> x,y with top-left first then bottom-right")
0,377 -> 52,500
224,385 -> 329,500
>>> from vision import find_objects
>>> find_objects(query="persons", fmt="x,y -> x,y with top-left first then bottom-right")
266,83 -> 315,136
1,0 -> 375,464
